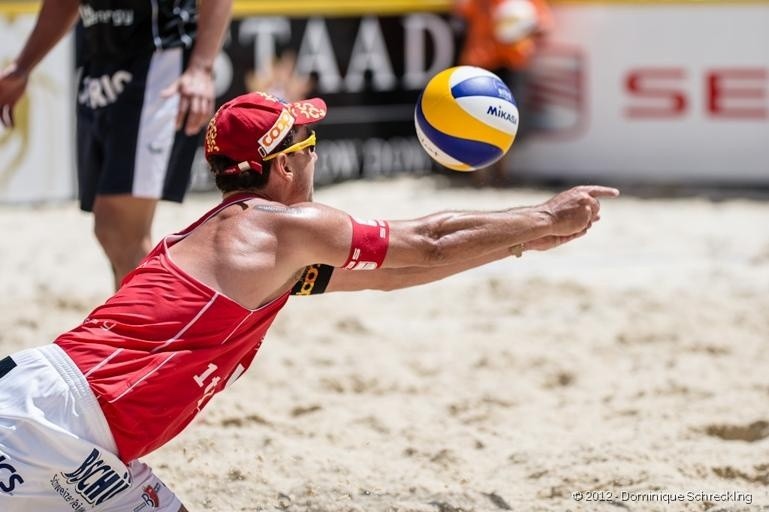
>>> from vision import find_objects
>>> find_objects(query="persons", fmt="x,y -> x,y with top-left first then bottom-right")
2,1 -> 241,301
0,87 -> 626,512
444,1 -> 554,190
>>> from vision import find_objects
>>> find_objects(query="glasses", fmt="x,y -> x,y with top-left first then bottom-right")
263,130 -> 317,163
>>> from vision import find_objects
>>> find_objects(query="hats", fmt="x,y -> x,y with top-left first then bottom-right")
204,90 -> 327,162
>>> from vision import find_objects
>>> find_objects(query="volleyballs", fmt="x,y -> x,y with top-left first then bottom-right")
414,65 -> 518,173
492,0 -> 536,43
